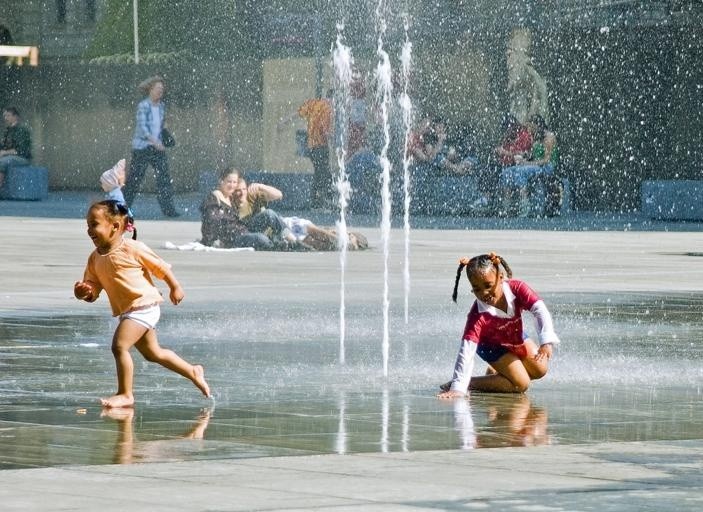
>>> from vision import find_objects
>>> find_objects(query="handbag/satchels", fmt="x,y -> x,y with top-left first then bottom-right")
161,127 -> 175,148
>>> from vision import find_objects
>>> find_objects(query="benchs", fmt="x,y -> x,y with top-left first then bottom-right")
0,163 -> 50,201
640,178 -> 701,222
350,172 -> 571,221
195,170 -> 318,212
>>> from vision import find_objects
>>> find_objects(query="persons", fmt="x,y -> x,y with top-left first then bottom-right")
440,392 -> 558,449
99,159 -> 135,223
0,107 -> 32,189
122,76 -> 182,217
232,178 -> 359,251
99,407 -> 213,465
201,167 -> 312,250
297,70 -> 560,219
74,200 -> 207,407
435,253 -> 558,399
